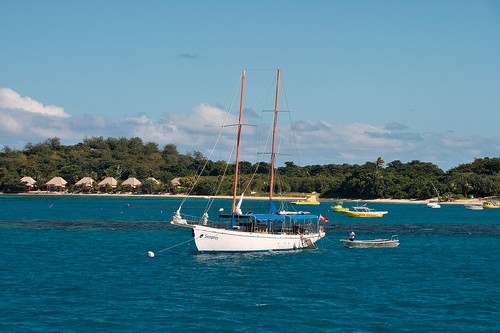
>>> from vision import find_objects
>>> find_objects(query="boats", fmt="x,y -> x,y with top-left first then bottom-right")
339,235 -> 401,249
427,203 -> 441,209
289,196 -> 320,205
466,203 -> 483,210
341,207 -> 388,218
332,205 -> 349,212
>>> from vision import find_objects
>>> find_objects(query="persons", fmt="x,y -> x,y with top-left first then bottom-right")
348,229 -> 355,241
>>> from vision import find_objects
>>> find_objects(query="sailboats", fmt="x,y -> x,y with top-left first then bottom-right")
171,70 -> 326,252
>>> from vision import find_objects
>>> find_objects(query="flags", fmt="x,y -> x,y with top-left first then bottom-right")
319,215 -> 328,222
